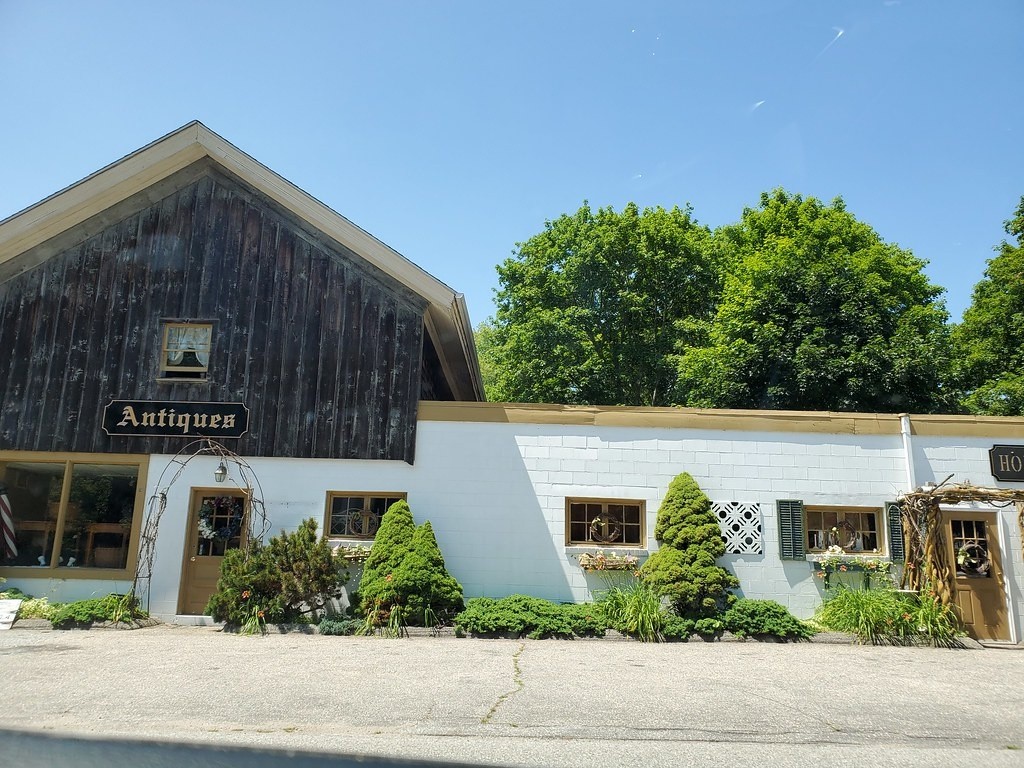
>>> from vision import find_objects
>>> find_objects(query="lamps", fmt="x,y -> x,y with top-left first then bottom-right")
59,556 -> 64,566
66,557 -> 76,567
214,462 -> 227,482
37,555 -> 46,566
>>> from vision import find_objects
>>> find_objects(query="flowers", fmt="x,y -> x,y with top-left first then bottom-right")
816,545 -> 899,578
198,496 -> 242,542
579,551 -> 640,573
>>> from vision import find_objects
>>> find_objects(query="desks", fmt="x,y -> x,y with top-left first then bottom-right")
15,520 -> 82,567
